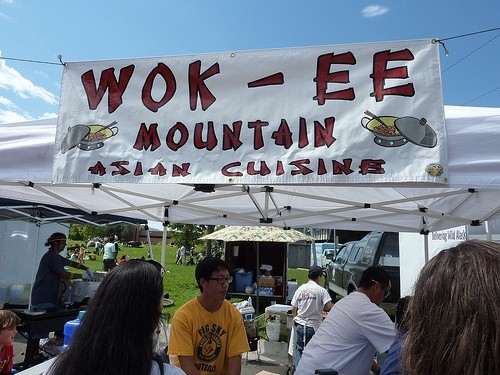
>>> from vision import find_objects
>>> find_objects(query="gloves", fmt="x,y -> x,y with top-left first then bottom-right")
81,268 -> 94,279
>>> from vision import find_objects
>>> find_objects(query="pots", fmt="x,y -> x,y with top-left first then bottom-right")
72,278 -> 102,303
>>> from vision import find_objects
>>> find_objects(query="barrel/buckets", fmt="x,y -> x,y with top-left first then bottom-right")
235,272 -> 253,292
264,304 -> 294,342
245,286 -> 253,293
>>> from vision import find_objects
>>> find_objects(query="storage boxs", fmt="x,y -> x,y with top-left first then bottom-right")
63,319 -> 81,345
239,305 -> 255,320
235,272 -> 254,291
287,280 -> 297,301
259,278 -> 275,287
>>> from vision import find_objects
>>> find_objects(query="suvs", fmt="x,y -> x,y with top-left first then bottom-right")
322,231 -> 400,323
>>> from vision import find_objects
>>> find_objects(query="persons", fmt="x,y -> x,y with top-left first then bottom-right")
0,310 -> 21,375
168,257 -> 251,375
379,239 -> 500,375
69,235 -> 128,272
31,232 -> 95,351
176,243 -> 224,265
291,266 -> 333,368
293,266 -> 397,375
46,258 -> 186,375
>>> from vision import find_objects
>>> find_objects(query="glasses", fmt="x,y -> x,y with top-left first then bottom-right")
209,276 -> 233,284
371,279 -> 391,298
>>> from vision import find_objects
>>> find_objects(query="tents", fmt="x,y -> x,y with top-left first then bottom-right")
0,104 -> 500,274
0,199 -> 155,337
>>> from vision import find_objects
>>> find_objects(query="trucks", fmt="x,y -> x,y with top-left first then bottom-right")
311,242 -> 339,261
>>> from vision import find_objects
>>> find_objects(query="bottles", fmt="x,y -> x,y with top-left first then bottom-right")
59,286 -> 72,303
255,287 -> 273,294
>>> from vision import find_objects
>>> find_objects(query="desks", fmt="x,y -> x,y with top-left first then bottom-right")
162,298 -> 174,343
13,308 -> 87,372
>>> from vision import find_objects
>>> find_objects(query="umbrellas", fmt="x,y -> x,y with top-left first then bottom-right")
197,226 -> 316,326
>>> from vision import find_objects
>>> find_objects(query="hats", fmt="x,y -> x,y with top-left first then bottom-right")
48,232 -> 67,242
309,266 -> 324,275
